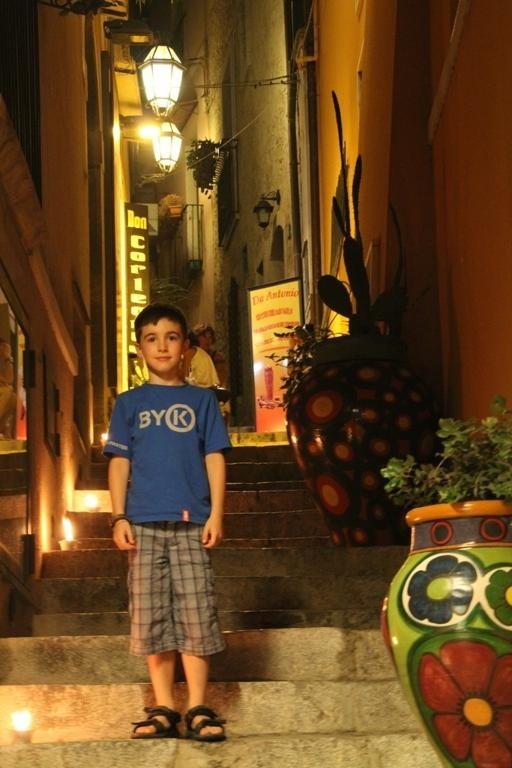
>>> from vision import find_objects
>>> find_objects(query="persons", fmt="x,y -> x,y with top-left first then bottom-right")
189,320 -> 226,386
183,327 -> 219,388
102,301 -> 234,740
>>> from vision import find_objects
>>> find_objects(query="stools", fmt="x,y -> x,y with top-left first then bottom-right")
211,387 -> 240,428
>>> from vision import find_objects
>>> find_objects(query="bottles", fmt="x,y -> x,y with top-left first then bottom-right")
263,366 -> 277,401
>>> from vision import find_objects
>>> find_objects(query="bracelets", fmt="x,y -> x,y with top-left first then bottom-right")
110,514 -> 132,526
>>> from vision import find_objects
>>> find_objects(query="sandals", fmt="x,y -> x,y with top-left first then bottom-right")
127,705 -> 180,741
183,705 -> 229,743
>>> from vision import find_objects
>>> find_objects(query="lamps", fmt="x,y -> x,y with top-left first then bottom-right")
254,189 -> 280,230
120,115 -> 184,176
107,0 -> 186,116
102,19 -> 156,48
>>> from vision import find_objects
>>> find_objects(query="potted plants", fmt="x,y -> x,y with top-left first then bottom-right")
256,87 -> 442,546
379,393 -> 512,767
154,192 -> 187,220
